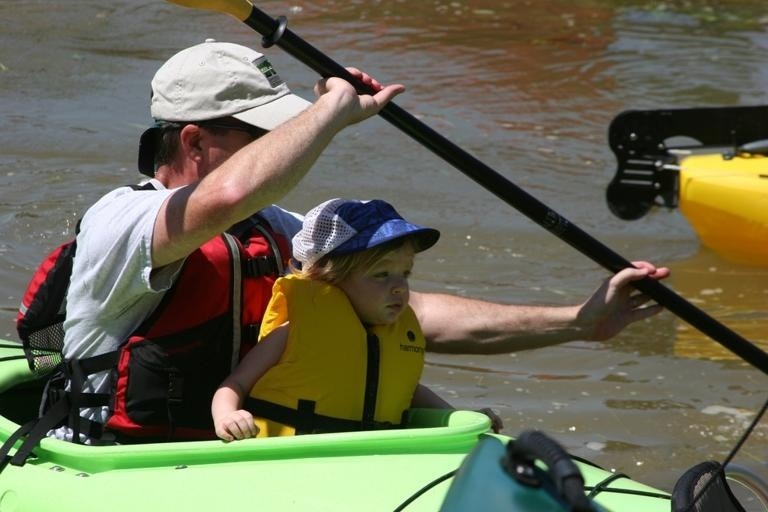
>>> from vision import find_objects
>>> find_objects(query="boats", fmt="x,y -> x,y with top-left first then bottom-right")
0,338 -> 680,512
675,152 -> 768,267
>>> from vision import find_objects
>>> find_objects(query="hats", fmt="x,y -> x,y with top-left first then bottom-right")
288,195 -> 440,274
147,37 -> 316,136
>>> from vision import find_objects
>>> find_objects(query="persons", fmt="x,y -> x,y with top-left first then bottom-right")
210,193 -> 444,443
41,34 -> 674,447
